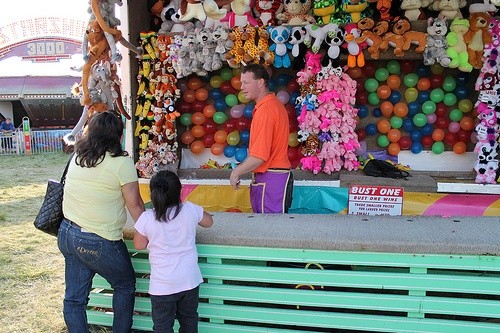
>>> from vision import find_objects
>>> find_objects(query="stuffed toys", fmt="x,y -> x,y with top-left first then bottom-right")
294,66 -> 364,176
472,66 -> 500,185
181,0 -> 500,67
62,0 -> 181,178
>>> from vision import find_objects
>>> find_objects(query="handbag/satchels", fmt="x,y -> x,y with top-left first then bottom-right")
34,149 -> 76,236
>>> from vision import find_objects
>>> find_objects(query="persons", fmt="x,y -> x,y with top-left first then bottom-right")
57,111 -> 146,333
230,64 -> 293,288
0,118 -> 15,152
133,169 -> 214,333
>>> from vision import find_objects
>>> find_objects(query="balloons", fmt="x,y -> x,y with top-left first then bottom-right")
177,67 -> 300,163
347,60 -> 478,156
